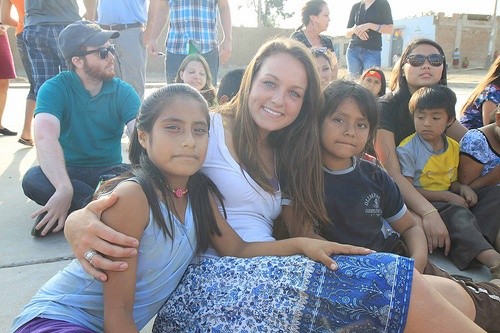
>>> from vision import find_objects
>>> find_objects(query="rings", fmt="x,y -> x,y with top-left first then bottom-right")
84,250 -> 97,262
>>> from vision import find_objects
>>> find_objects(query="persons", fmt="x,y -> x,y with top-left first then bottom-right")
290,0 -> 334,52
346,0 -> 394,80
0,0 -> 96,147
9,83 -> 377,333
64,38 -> 490,333
95,0 -> 156,139
148,0 -> 231,86
22,20 -> 500,280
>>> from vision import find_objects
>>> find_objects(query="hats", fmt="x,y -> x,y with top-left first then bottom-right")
58,20 -> 120,59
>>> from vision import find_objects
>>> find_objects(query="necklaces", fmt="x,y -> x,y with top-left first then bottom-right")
165,180 -> 189,198
260,157 -> 275,178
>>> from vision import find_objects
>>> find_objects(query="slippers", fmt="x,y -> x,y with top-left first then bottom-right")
19,137 -> 35,147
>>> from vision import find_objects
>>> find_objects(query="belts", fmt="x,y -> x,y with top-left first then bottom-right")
99,23 -> 143,30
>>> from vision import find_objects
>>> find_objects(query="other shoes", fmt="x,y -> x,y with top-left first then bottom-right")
30,210 -> 71,236
0,127 -> 17,135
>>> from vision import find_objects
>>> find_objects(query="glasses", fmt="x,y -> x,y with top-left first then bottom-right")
79,43 -> 116,60
310,48 -> 327,54
402,53 -> 445,67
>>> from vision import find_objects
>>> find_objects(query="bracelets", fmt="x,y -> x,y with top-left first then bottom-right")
422,209 -> 437,218
376,24 -> 382,33
